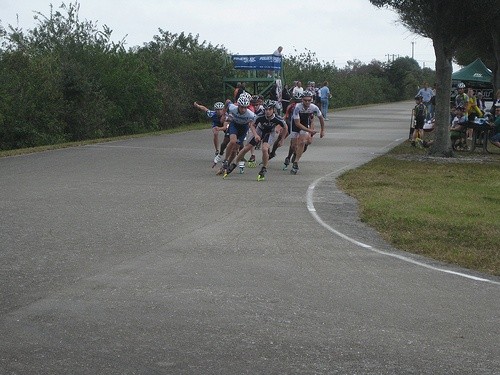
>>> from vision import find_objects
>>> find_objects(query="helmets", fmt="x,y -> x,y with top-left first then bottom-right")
252,95 -> 259,101
237,97 -> 250,106
263,100 -> 276,109
240,93 -> 252,100
214,102 -> 224,109
414,93 -> 423,99
457,82 -> 465,88
301,91 -> 313,97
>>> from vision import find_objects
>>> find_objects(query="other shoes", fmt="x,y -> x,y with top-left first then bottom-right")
424,119 -> 426,121
428,120 -> 431,124
323,118 -> 329,121
411,141 -> 416,148
416,138 -> 428,148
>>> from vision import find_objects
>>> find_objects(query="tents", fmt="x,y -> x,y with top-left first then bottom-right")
451,58 -> 493,83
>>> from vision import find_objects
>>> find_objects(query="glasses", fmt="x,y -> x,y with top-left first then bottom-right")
303,99 -> 312,102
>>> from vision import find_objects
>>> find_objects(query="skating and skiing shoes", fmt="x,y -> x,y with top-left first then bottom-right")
283,157 -> 291,170
257,166 -> 268,181
259,152 -> 275,165
255,141 -> 261,149
215,161 -> 229,175
291,162 -> 298,174
223,162 -> 237,178
248,155 -> 256,167
238,160 -> 245,174
212,153 -> 222,169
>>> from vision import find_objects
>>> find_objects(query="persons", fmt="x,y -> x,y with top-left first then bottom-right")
409,94 -> 427,147
194,81 -> 332,181
222,100 -> 289,181
282,90 -> 325,175
409,82 -> 500,153
266,47 -> 283,78
